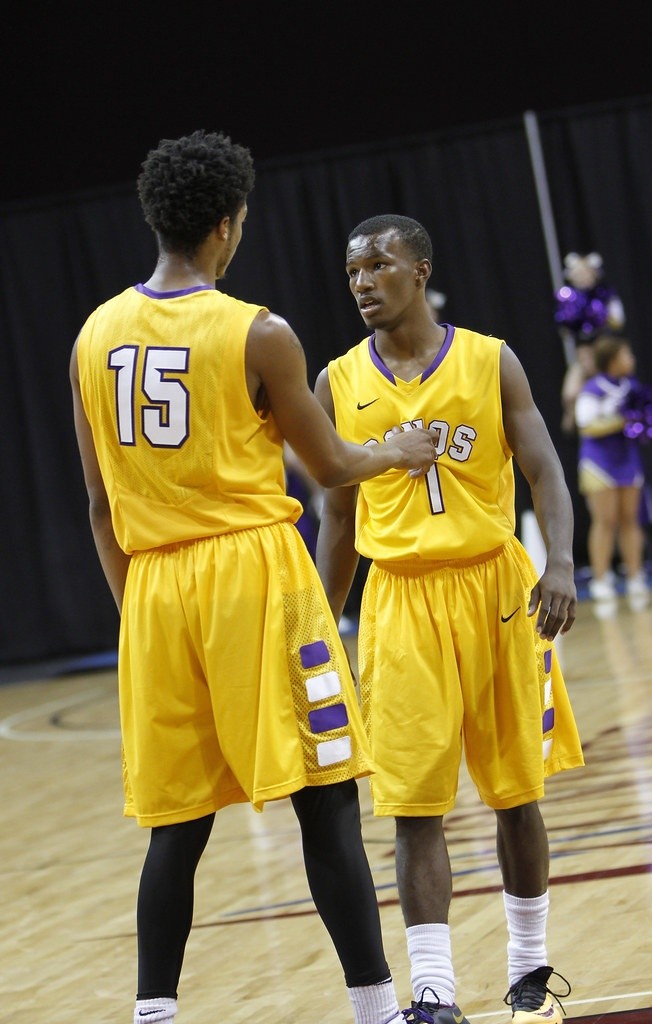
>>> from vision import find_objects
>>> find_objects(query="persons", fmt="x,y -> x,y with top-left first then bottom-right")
284,215 -> 652,1024
70,130 -> 438,1024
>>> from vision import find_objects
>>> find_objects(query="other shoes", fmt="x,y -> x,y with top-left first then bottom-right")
591,578 -> 618,617
627,569 -> 650,610
386,1012 -> 406,1024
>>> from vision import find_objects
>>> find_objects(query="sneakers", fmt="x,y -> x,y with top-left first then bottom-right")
503,966 -> 571,1024
400,986 -> 470,1024
